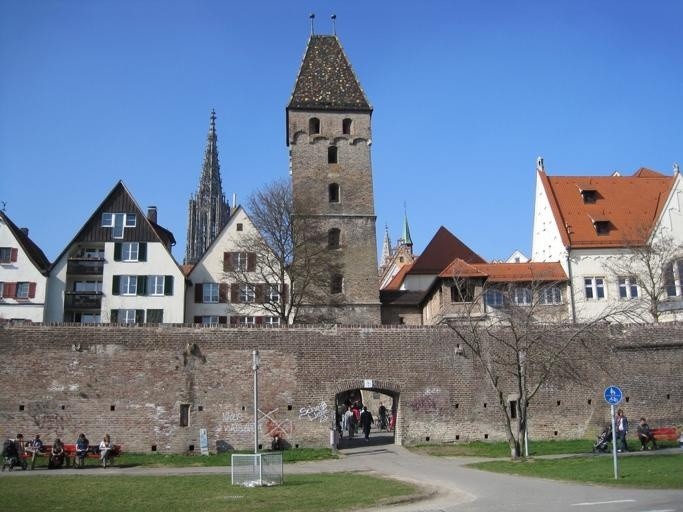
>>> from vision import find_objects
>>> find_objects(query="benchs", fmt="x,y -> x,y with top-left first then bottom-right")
15,444 -> 123,469
637,428 -> 679,450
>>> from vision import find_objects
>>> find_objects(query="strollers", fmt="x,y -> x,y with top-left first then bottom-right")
591,422 -> 611,452
2,440 -> 28,471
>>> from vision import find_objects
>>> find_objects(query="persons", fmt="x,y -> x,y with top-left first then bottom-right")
48,438 -> 65,467
99,434 -> 114,469
609,410 -> 629,453
636,418 -> 657,451
379,401 -> 388,424
13,434 -> 48,470
341,393 -> 374,442
76,433 -> 89,468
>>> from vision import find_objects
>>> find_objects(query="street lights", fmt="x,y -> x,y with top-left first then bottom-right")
251,345 -> 259,475
518,346 -> 528,457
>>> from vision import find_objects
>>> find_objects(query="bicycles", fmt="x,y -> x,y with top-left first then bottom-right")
376,414 -> 391,432
